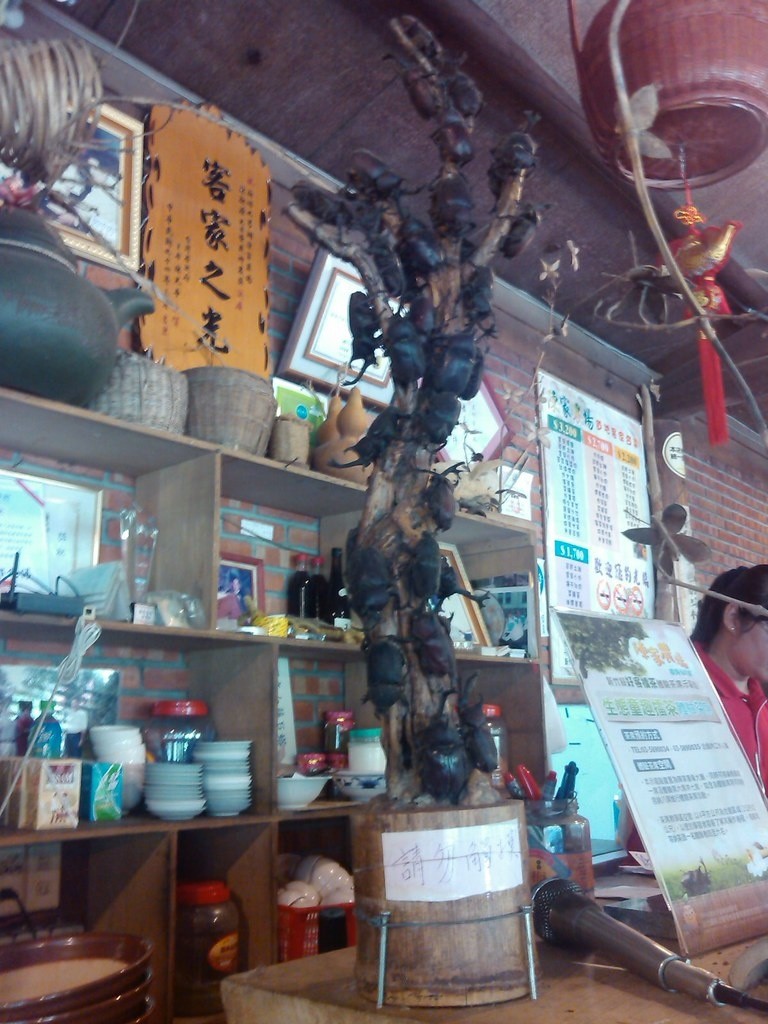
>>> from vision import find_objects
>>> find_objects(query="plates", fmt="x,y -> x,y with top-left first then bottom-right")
142,741 -> 253,820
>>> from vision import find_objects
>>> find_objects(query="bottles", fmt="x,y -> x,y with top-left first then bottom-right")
288,547 -> 351,632
27,701 -> 62,757
12,701 -> 35,756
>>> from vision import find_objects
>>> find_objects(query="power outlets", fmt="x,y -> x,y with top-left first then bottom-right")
0,844 -> 26,918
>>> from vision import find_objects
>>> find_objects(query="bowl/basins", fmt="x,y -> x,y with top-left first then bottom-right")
333,771 -> 386,804
0,941 -> 165,1024
277,776 -> 333,808
89,725 -> 146,816
276,854 -> 354,908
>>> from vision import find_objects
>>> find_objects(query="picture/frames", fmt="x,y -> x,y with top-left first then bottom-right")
215,551 -> 266,632
0,102 -> 143,273
434,540 -> 493,652
274,245 -> 421,410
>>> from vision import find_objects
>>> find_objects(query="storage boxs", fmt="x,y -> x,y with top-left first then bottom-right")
0,756 -> 125,832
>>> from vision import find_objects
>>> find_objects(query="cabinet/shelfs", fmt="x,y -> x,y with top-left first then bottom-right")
0,385 -> 545,1024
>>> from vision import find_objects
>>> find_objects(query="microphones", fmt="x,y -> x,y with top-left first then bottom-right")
530,875 -> 749,1007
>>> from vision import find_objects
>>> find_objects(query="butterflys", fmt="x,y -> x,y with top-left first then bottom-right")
619,502 -> 713,576
613,83 -> 673,160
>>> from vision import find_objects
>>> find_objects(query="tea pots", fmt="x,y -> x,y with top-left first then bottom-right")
1,205 -> 155,407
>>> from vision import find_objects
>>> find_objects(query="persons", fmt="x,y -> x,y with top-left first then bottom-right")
622,563 -> 768,867
228,577 -> 247,610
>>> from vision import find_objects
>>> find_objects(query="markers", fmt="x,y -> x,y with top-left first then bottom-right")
541,771 -> 557,800
518,764 -> 541,800
555,761 -> 579,800
504,773 -> 524,799
